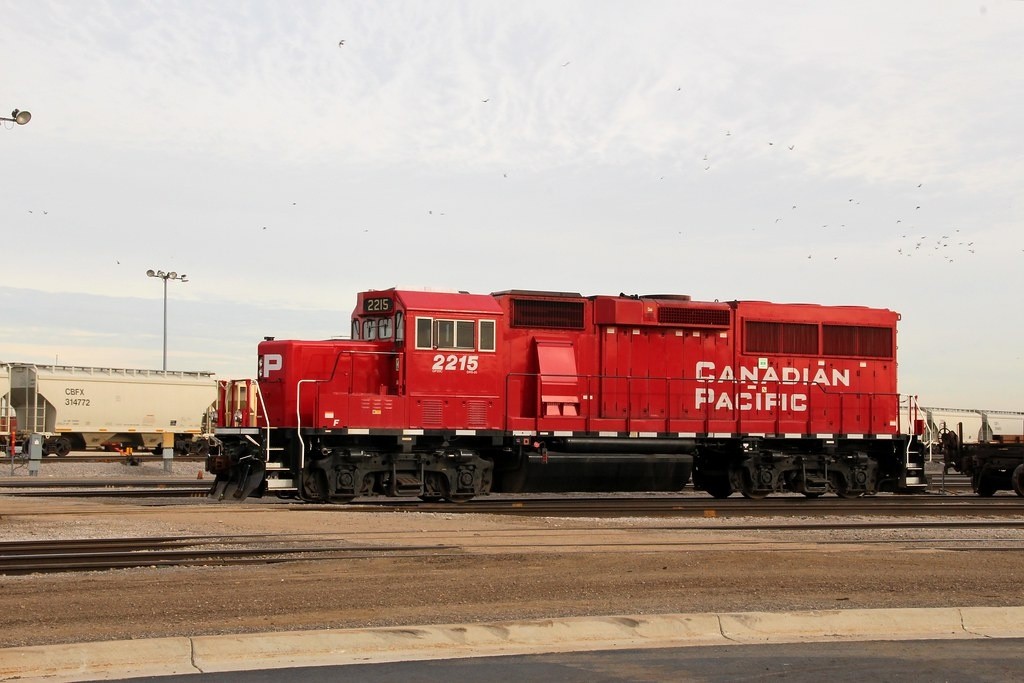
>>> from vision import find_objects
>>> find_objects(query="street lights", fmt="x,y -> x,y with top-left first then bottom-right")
147,269 -> 189,371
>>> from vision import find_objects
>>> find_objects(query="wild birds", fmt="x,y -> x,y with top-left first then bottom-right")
482,98 -> 488,102
117,261 -> 120,264
776,183 -> 975,265
562,61 -> 570,66
703,131 -> 795,170
428,210 -> 443,214
30,210 -> 48,215
677,87 -> 679,91
339,39 -> 344,48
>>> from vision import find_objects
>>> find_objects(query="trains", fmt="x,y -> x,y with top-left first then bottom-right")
202,287 -> 931,503
0,360 -> 217,457
937,428 -> 1024,498
